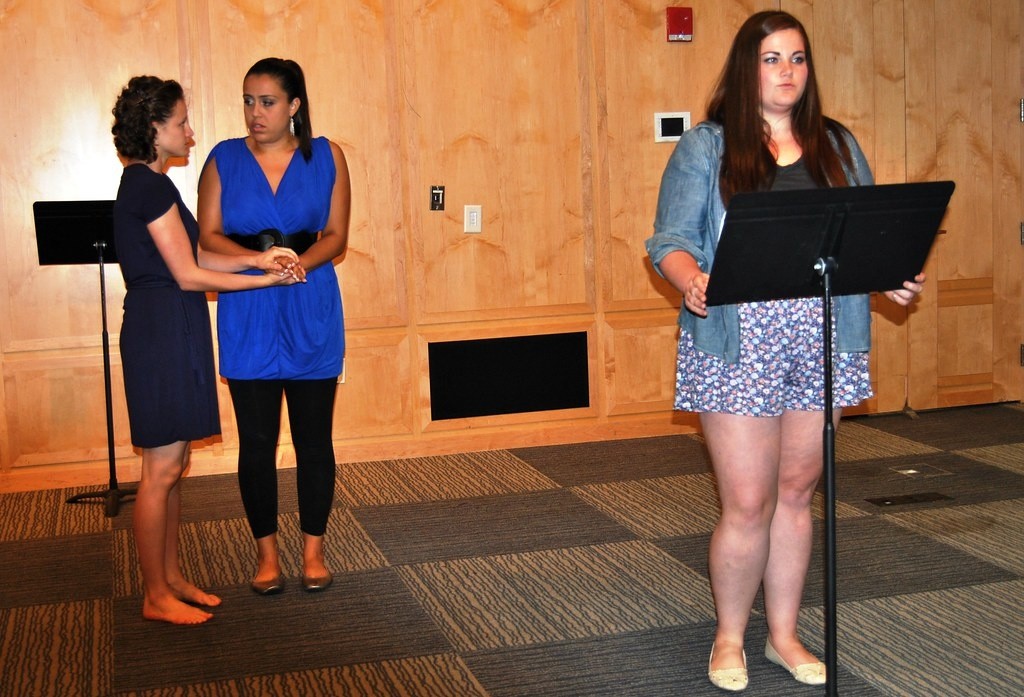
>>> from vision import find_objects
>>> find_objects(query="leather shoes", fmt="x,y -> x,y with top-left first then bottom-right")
707,634 -> 749,693
764,632 -> 827,685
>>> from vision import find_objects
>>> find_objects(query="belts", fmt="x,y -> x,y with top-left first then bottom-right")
225,229 -> 318,256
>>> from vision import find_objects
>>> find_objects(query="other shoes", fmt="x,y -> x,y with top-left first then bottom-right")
252,553 -> 286,595
301,559 -> 333,592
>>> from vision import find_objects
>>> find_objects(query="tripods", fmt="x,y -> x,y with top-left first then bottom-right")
33,201 -> 140,518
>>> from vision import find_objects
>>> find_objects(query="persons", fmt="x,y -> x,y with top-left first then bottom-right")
198,58 -> 350,594
645,10 -> 925,690
113,75 -> 298,623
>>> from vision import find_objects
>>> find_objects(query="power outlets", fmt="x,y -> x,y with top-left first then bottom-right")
464,204 -> 482,233
